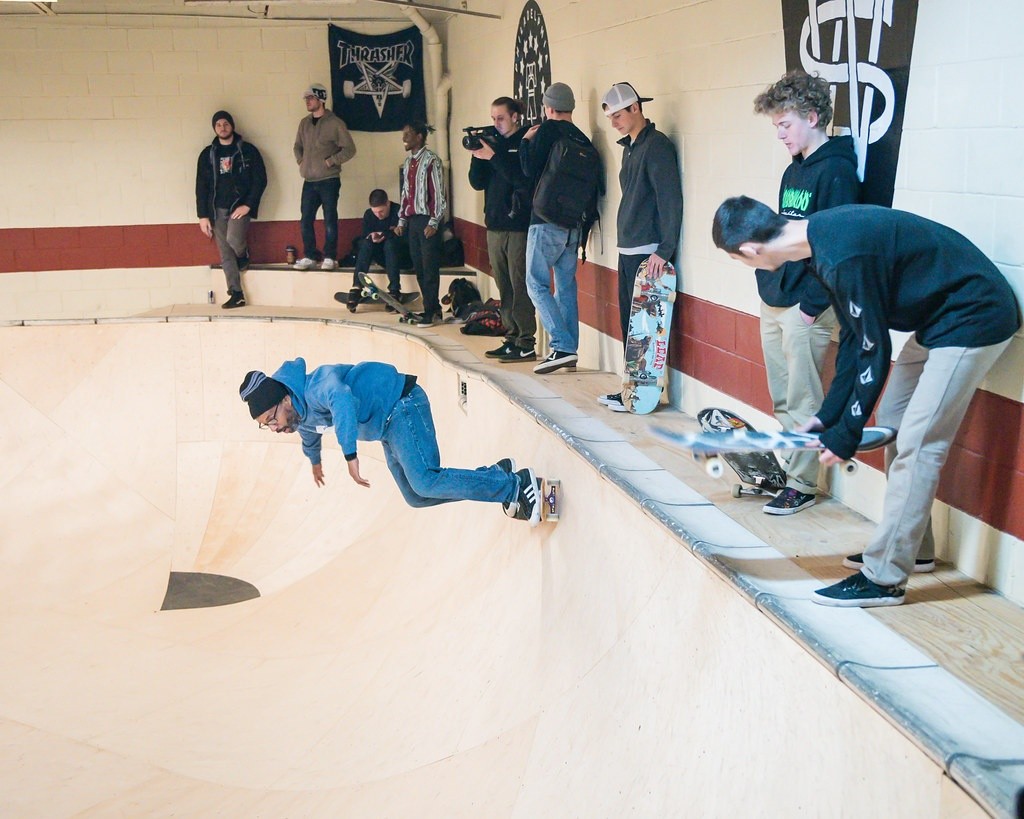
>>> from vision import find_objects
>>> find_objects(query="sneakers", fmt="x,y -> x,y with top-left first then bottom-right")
498,346 -> 537,362
484,341 -> 515,358
557,362 -> 576,372
533,350 -> 578,374
417,312 -> 442,327
596,391 -> 624,405
841,552 -> 936,573
608,399 -> 660,412
810,570 -> 906,607
515,467 -> 541,526
763,487 -> 816,515
496,457 -> 517,518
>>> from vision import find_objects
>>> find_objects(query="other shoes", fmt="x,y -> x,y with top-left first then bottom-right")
346,288 -> 364,309
221,291 -> 245,308
321,258 -> 338,270
384,291 -> 401,311
237,244 -> 251,271
293,257 -> 317,269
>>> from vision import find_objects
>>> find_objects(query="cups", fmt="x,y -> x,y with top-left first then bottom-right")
286,246 -> 295,265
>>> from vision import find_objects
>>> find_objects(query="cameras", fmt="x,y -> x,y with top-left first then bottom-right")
462,126 -> 504,150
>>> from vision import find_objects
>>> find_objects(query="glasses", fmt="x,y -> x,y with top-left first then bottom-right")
259,401 -> 278,430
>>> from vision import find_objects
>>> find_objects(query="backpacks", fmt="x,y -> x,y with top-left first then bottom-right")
460,297 -> 509,336
531,120 -> 601,232
449,279 -> 482,317
441,222 -> 464,267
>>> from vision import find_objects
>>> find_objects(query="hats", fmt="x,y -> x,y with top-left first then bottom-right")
601,82 -> 654,116
304,83 -> 327,102
212,110 -> 235,130
542,82 -> 576,112
239,370 -> 289,420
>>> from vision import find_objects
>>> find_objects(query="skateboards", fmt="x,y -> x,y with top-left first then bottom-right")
619,256 -> 678,416
500,469 -> 562,524
696,405 -> 788,497
640,416 -> 898,478
333,290 -> 421,314
357,271 -> 424,326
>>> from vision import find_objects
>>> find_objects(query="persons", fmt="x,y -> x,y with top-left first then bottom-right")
468,94 -> 540,364
754,66 -> 864,515
598,82 -> 686,413
236,356 -> 541,528
195,110 -> 270,310
347,186 -> 406,310
518,79 -> 605,374
393,120 -> 450,328
710,192 -> 1022,608
292,81 -> 358,273
